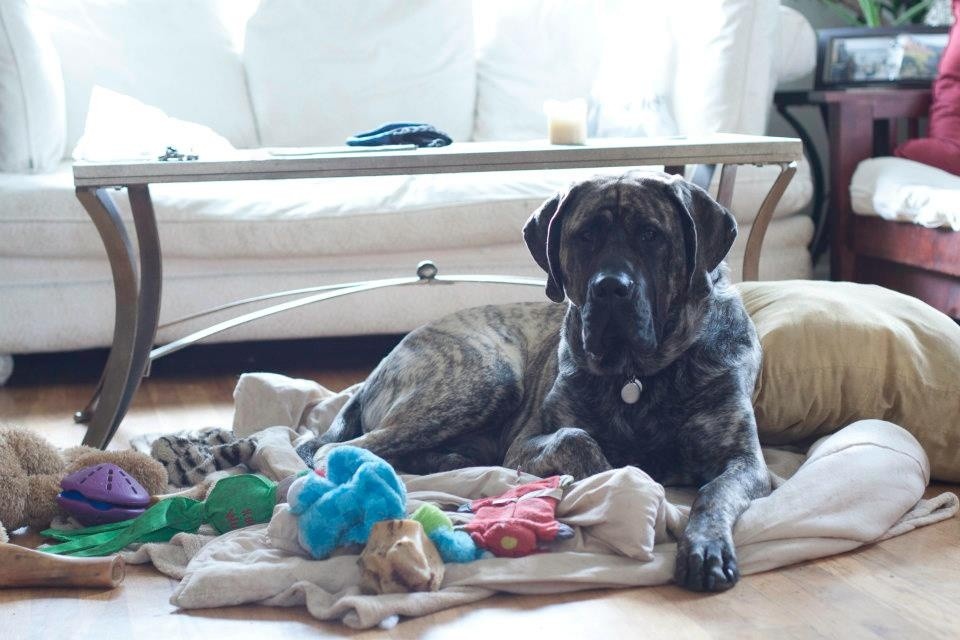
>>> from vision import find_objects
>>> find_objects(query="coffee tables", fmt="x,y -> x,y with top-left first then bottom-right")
71,131 -> 810,440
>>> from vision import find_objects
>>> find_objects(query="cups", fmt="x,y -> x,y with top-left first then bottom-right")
548,96 -> 590,148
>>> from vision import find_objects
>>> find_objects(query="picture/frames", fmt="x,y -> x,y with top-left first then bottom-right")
810,17 -> 952,86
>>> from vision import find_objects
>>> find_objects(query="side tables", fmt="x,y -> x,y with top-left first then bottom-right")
768,84 -> 934,289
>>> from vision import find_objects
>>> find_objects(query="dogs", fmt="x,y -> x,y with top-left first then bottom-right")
296,172 -> 772,592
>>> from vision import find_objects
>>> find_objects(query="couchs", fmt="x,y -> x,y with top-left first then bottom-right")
0,0 -> 821,365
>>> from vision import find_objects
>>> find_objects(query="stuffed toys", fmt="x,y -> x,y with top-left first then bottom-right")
287,445 -> 476,564
0,425 -> 168,532
151,428 -> 258,489
